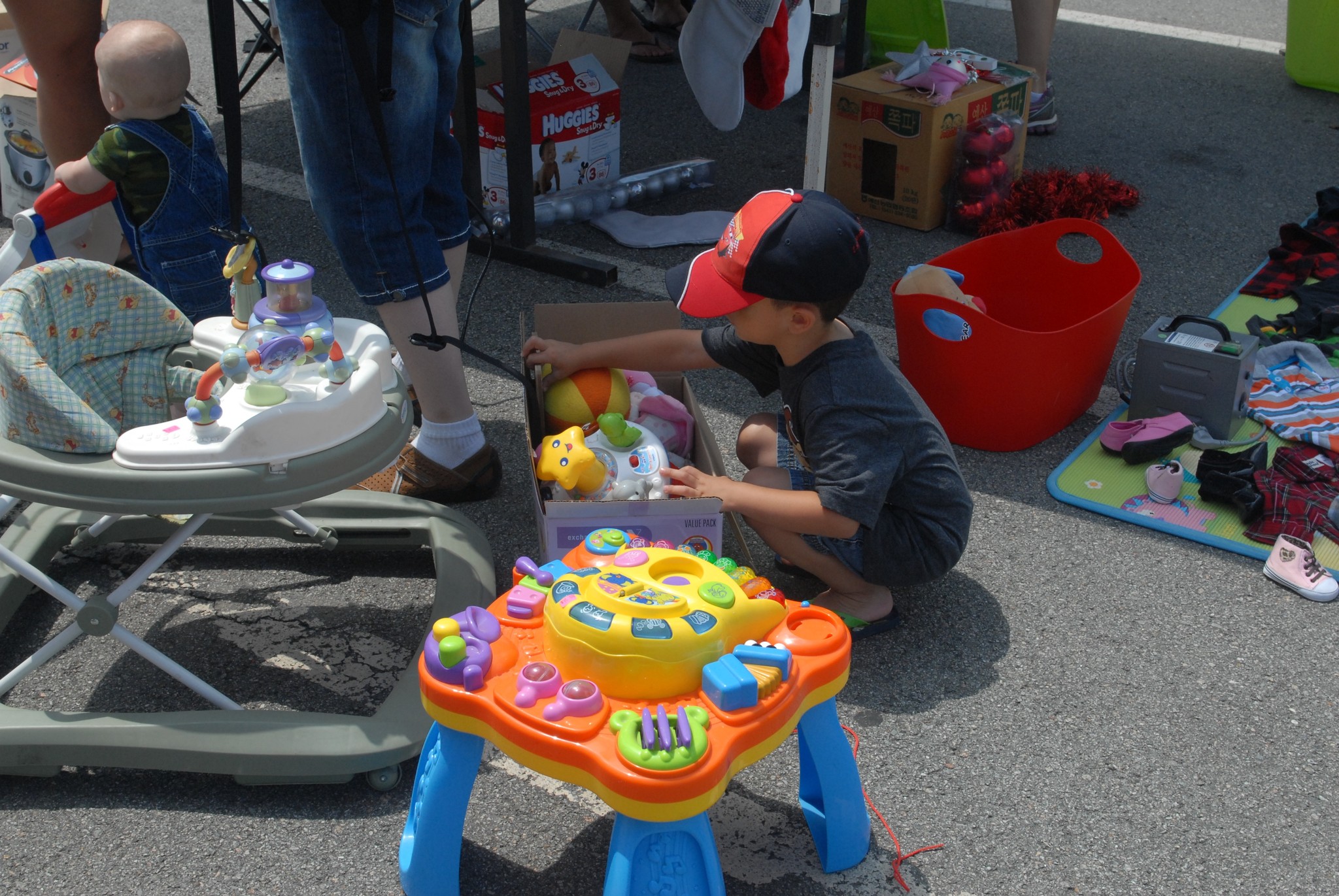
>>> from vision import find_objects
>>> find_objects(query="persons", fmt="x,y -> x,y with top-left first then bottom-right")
1005,0 -> 1058,136
268,0 -> 503,503
520,188 -> 972,643
54,21 -> 265,326
598,0 -> 690,62
2,0 -> 112,169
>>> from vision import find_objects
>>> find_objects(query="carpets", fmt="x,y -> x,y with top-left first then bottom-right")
1046,206 -> 1339,584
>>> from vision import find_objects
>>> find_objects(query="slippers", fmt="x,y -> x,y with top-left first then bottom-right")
626,37 -> 677,63
806,595 -> 895,640
652,18 -> 687,37
775,552 -> 813,576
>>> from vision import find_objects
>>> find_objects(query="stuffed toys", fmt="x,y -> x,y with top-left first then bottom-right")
623,369 -> 695,456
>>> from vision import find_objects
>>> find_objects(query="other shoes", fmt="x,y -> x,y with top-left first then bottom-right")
1026,71 -> 1057,135
353,442 -> 502,501
1122,412 -> 1192,466
1146,461 -> 1185,504
1264,534 -> 1338,601
1099,417 -> 1145,454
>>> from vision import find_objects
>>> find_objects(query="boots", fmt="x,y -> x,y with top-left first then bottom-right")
1195,442 -> 1269,480
1198,470 -> 1264,524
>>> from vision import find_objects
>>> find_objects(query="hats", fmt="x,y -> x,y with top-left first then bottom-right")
665,188 -> 871,319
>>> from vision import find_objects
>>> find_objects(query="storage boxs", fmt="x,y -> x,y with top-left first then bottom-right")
0,54 -> 55,220
519,302 -> 759,575
476,28 -> 632,208
825,61 -> 1036,231
